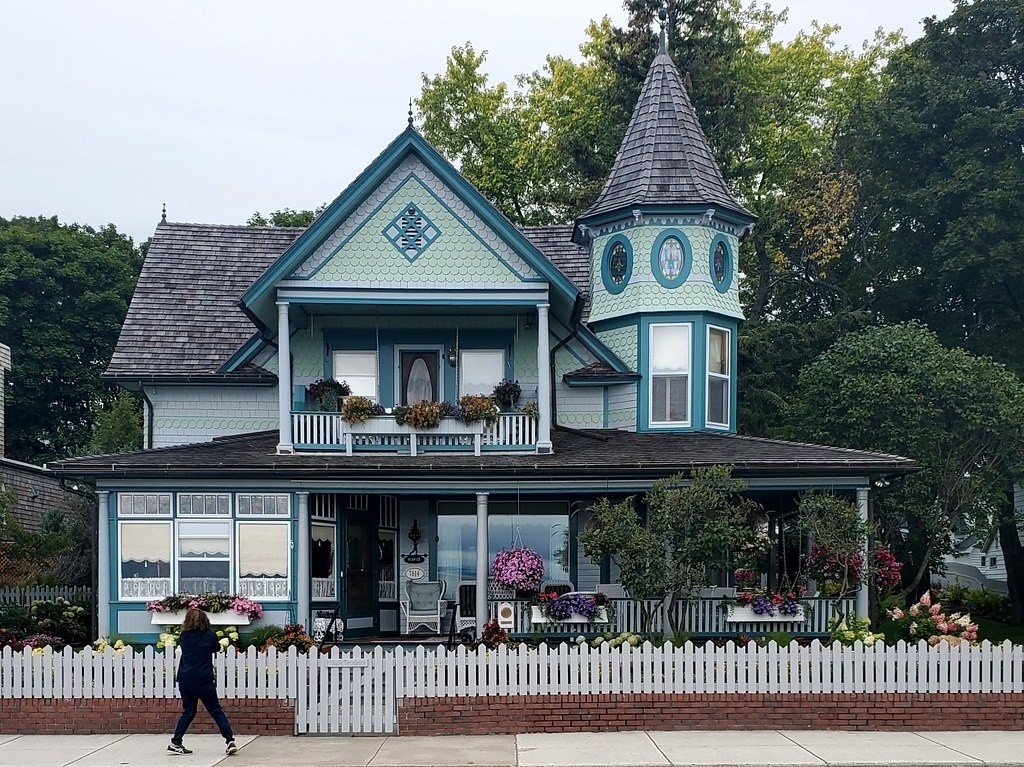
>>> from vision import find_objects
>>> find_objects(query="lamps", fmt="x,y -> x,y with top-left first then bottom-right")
448,345 -> 456,367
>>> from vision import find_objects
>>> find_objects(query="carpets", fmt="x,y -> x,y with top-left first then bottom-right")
426,637 -> 463,643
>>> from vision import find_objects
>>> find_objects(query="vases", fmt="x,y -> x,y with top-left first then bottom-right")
151,608 -> 252,625
530,606 -> 608,623
343,415 -> 483,437
313,618 -> 344,643
726,605 -> 805,621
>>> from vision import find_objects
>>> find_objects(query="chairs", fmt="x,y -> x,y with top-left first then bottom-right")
454,580 -> 477,636
595,584 -> 630,598
541,580 -> 574,596
693,585 -> 820,624
400,580 -> 448,638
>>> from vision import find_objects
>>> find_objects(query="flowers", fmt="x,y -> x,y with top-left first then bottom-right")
317,611 -> 341,618
491,546 -> 545,591
802,542 -> 903,587
522,591 -> 616,631
305,376 -> 540,429
734,568 -> 753,582
146,590 -> 263,621
717,586 -> 808,618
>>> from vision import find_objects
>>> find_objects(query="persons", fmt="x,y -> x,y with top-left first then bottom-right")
165,608 -> 237,756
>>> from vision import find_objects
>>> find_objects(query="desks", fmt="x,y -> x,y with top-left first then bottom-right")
487,599 -> 528,633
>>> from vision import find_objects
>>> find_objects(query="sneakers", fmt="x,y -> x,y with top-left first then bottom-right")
225,741 -> 238,755
165,740 -> 193,756
